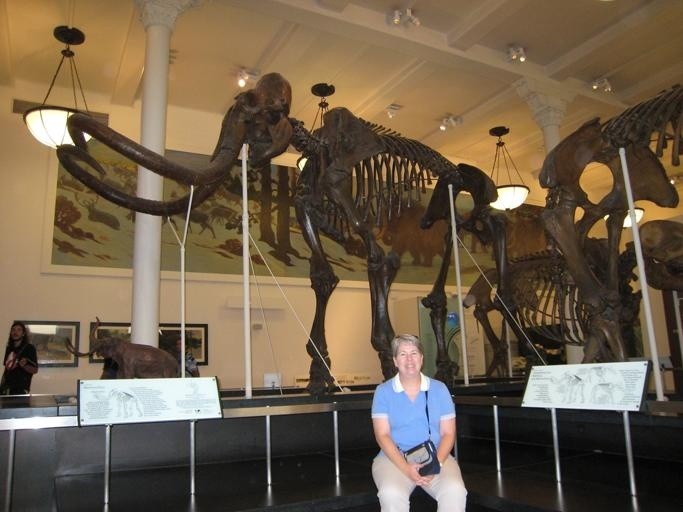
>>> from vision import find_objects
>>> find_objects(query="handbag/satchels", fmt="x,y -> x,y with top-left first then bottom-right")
404,441 -> 440,475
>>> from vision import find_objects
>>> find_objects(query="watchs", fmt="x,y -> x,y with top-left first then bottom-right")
438,460 -> 442,469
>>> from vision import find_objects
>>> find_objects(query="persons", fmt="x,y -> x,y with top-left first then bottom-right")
172,340 -> 198,377
97,348 -> 117,380
368,332 -> 468,512
0,321 -> 39,395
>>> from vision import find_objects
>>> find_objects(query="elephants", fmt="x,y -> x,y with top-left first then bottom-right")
65,317 -> 181,380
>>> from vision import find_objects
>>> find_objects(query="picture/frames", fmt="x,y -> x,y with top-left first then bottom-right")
37,138 -> 555,299
12,319 -> 209,368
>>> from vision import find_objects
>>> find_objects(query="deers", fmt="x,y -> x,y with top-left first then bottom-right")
74,192 -> 121,230
